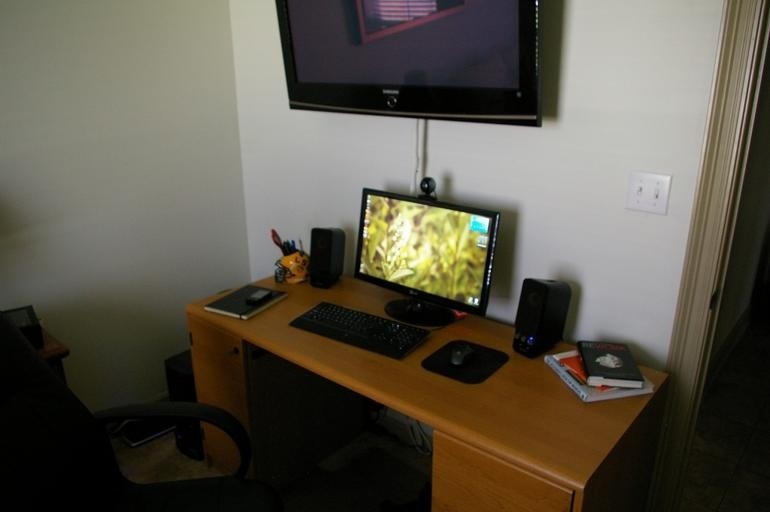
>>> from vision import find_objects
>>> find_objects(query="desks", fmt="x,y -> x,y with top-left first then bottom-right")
181,270 -> 671,512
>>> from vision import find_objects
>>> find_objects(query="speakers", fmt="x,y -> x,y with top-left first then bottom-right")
512,278 -> 572,359
307,228 -> 345,289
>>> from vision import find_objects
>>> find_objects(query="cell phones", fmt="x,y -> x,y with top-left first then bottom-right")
245,287 -> 273,307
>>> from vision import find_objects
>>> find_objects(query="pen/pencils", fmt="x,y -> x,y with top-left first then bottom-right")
283,240 -> 296,254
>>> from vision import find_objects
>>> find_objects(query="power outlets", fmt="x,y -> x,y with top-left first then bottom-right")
625,171 -> 671,214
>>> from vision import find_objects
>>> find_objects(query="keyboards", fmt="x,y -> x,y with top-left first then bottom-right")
289,301 -> 432,361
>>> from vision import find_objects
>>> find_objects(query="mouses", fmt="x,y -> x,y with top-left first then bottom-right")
450,344 -> 471,367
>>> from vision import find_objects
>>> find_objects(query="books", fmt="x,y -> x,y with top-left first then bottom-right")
203,285 -> 289,321
542,340 -> 654,403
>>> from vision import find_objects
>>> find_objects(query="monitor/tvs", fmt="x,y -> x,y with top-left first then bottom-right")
354,188 -> 500,327
276,0 -> 542,128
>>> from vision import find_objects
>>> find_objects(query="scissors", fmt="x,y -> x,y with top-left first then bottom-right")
272,229 -> 287,256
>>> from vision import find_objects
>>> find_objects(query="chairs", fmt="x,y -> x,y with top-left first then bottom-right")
0,316 -> 314,511
